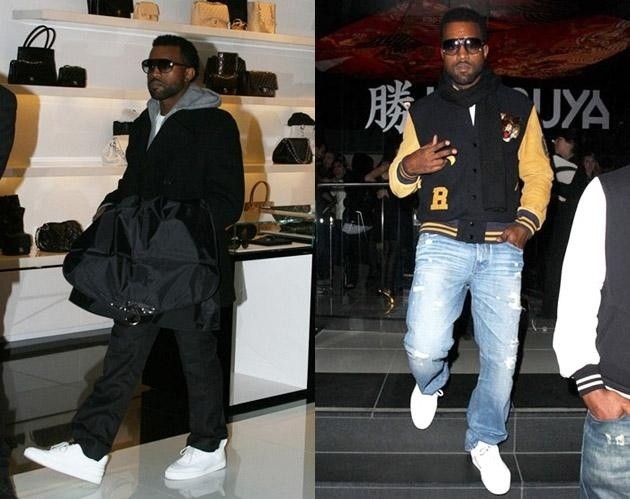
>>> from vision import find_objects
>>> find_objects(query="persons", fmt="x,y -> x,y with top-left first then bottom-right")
543,137 -> 616,179
553,164 -> 630,499
23,35 -> 244,485
315,145 -> 399,277
388,8 -> 554,495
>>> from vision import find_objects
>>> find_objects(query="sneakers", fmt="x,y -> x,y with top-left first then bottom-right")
24,442 -> 110,486
470,440 -> 511,495
410,384 -> 444,430
165,438 -> 228,481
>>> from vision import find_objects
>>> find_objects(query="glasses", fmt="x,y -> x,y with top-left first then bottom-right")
442,37 -> 486,56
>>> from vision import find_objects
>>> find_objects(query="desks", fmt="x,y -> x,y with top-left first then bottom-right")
316,183 -> 390,296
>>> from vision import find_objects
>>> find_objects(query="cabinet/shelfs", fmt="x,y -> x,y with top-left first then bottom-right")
1,9 -> 315,448
